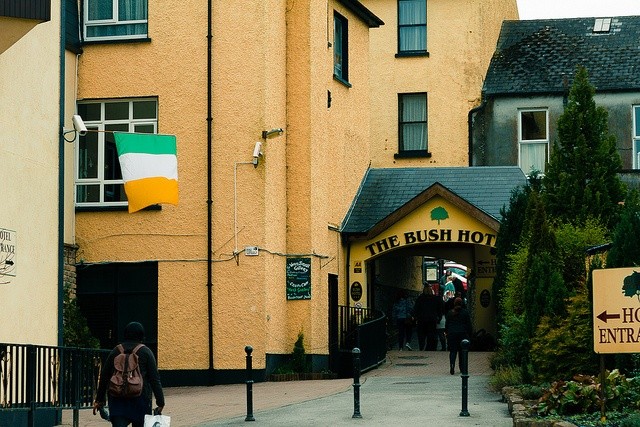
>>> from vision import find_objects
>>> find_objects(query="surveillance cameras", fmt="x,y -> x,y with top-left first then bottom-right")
72,115 -> 87,135
253,142 -> 262,158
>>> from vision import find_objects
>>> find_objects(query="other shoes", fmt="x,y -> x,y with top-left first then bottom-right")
440,349 -> 446,351
450,364 -> 454,375
420,347 -> 423,351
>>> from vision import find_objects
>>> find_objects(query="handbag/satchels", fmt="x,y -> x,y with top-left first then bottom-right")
144,410 -> 171,427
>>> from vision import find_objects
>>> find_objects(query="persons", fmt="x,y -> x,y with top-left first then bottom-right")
452,276 -> 465,299
95,321 -> 165,427
444,297 -> 473,375
443,276 -> 455,302
439,269 -> 452,297
412,285 -> 442,351
435,314 -> 446,351
392,289 -> 415,352
445,290 -> 466,318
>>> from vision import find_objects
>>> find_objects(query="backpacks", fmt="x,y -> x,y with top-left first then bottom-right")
109,344 -> 145,398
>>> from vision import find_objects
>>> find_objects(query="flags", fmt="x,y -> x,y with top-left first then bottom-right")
113,131 -> 180,214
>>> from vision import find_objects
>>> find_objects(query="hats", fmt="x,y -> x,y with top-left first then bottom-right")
454,298 -> 463,305
125,322 -> 145,340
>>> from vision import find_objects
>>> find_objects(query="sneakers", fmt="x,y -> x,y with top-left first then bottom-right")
405,342 -> 412,351
399,348 -> 403,351
